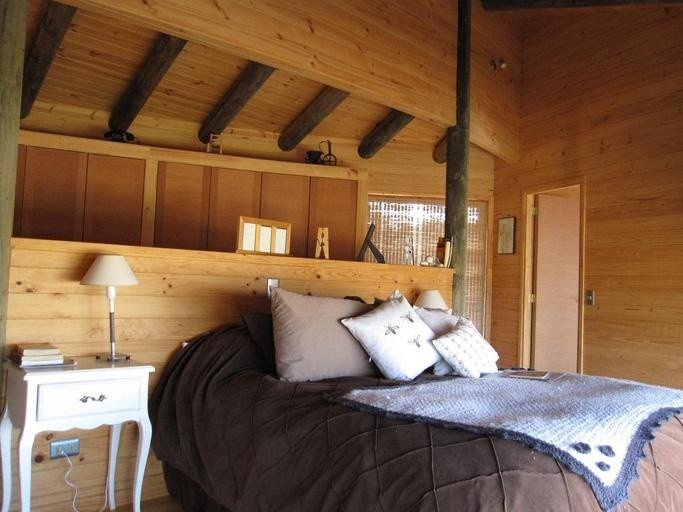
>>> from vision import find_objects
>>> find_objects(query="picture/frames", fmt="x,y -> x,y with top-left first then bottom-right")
356,222 -> 386,263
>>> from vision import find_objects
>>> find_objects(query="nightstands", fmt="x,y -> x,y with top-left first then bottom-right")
0,351 -> 157,512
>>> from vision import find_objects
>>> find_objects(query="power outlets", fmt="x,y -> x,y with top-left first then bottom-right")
49,435 -> 79,461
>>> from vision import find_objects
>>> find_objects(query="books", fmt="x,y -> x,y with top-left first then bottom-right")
438,236 -> 453,267
508,371 -> 549,380
478,370 -> 568,382
8,342 -> 78,369
435,241 -> 450,268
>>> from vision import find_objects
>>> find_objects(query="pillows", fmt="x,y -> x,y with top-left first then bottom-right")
241,285 -> 500,383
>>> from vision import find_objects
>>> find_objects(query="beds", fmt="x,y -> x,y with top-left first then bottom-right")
147,308 -> 682,510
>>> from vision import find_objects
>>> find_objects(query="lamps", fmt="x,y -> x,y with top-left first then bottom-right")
490,56 -> 508,71
77,254 -> 139,361
